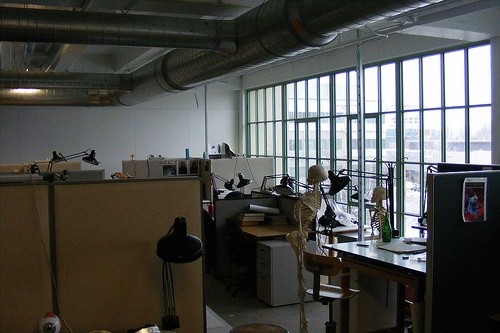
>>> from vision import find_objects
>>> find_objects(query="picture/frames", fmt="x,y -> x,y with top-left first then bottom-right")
176,160 -> 200,175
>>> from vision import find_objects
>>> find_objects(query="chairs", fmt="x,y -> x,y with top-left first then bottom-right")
304,251 -> 363,333
224,218 -> 257,298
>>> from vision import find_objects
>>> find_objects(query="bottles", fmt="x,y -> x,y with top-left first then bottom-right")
382,213 -> 392,242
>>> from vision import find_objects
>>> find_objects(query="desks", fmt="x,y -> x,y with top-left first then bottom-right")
240,222 -> 427,333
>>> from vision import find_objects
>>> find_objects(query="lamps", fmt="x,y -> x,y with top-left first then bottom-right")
210,167 -> 400,238
30,148 -> 99,181
156,217 -> 203,331
